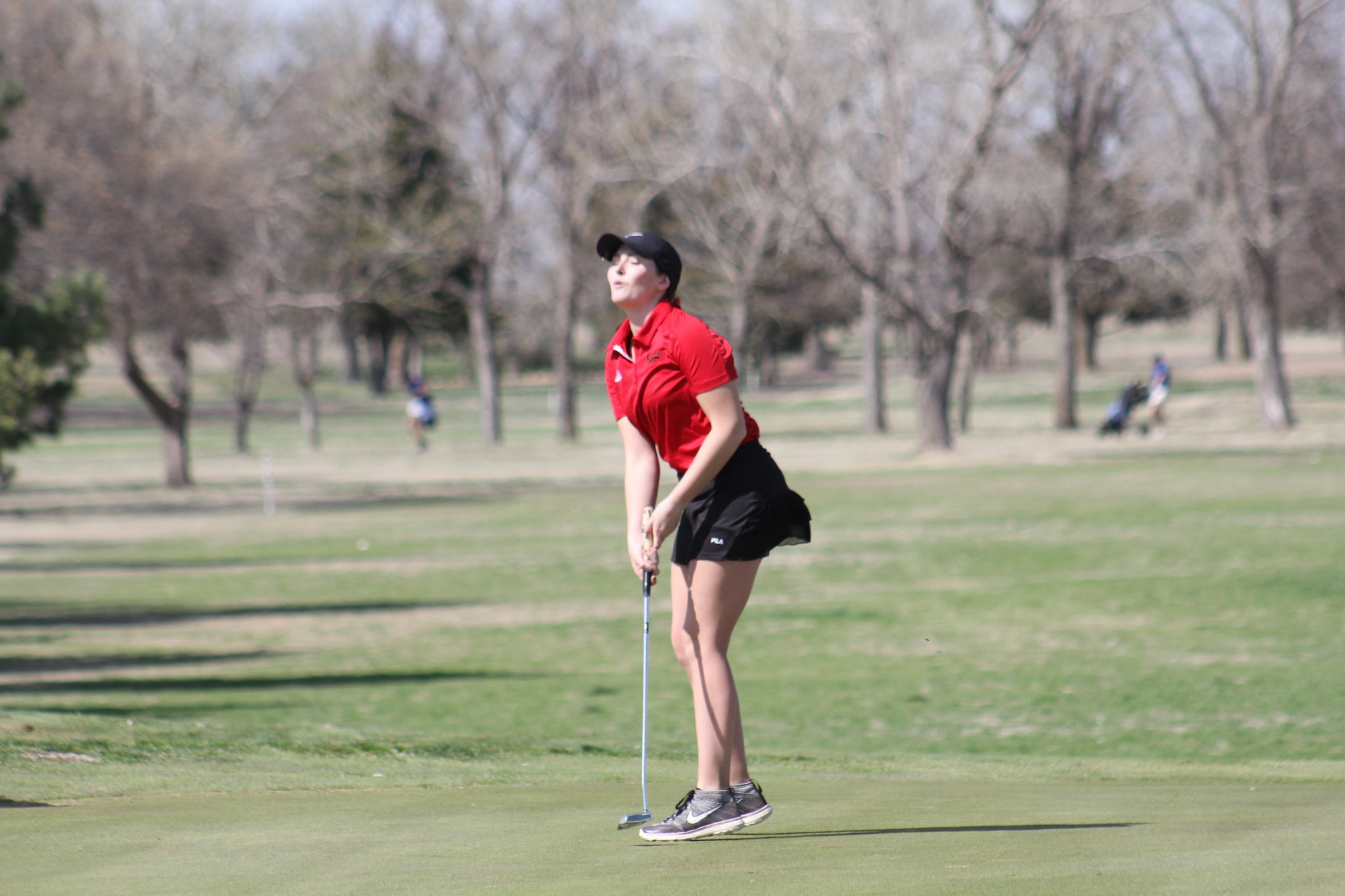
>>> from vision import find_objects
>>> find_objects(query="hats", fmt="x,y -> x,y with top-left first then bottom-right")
597,232 -> 682,284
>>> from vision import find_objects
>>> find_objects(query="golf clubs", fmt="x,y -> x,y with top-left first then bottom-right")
614,504 -> 655,831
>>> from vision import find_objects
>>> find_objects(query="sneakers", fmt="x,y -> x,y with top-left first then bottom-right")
729,779 -> 773,826
639,787 -> 744,842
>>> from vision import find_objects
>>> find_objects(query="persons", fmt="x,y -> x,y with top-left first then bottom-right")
1143,352 -> 1173,441
403,365 -> 436,445
593,230 -> 811,840
